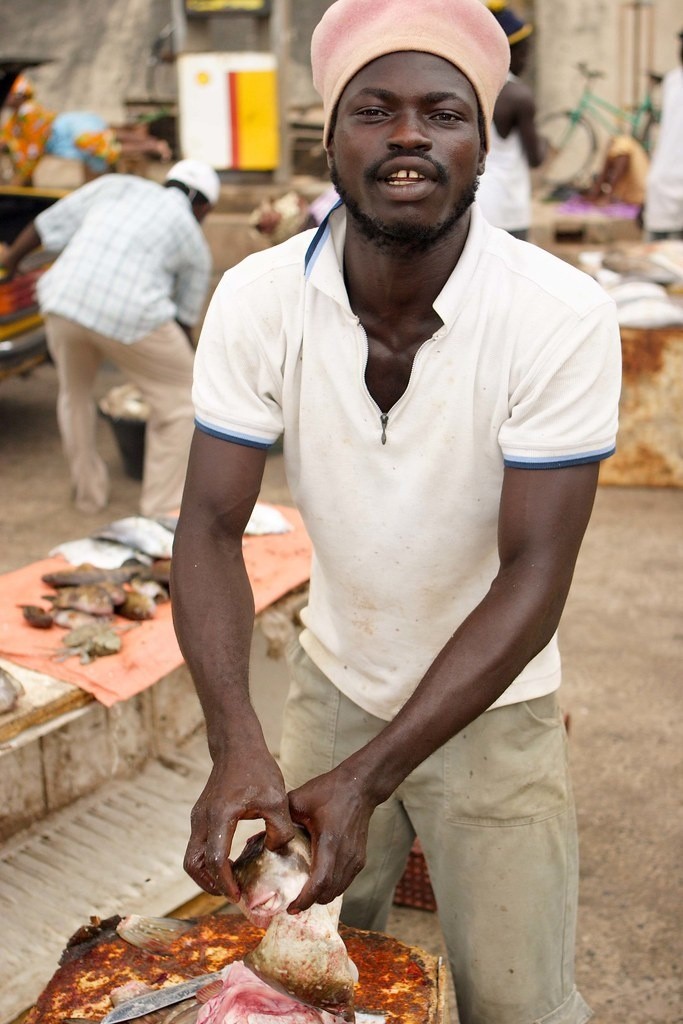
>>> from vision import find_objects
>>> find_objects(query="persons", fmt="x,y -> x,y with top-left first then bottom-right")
474,0 -> 542,241
643,31 -> 683,245
167,0 -> 624,1024
0,159 -> 224,539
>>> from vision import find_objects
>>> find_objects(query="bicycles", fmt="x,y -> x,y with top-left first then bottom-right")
532,60 -> 664,200
